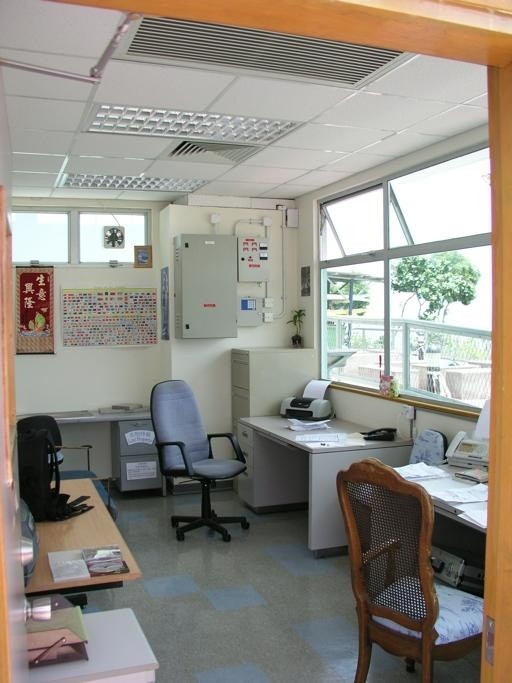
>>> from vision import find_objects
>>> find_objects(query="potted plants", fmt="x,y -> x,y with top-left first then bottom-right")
287,307 -> 307,346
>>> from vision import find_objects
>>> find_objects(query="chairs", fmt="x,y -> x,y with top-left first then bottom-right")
334,456 -> 484,683
149,378 -> 250,542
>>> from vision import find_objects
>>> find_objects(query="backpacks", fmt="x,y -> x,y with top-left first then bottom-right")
17,415 -> 70,522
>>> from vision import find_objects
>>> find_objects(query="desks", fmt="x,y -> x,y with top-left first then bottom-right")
14,406 -> 161,682
390,463 -> 485,567
235,415 -> 414,561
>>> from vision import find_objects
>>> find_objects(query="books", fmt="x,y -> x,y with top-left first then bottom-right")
48,550 -> 91,583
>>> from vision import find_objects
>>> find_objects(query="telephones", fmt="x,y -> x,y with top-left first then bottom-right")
364,428 -> 397,441
445,430 -> 489,469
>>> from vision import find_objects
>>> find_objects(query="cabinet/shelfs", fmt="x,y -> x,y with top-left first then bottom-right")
230,346 -> 315,492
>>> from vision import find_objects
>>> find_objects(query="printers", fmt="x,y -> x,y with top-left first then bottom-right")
279,397 -> 332,421
